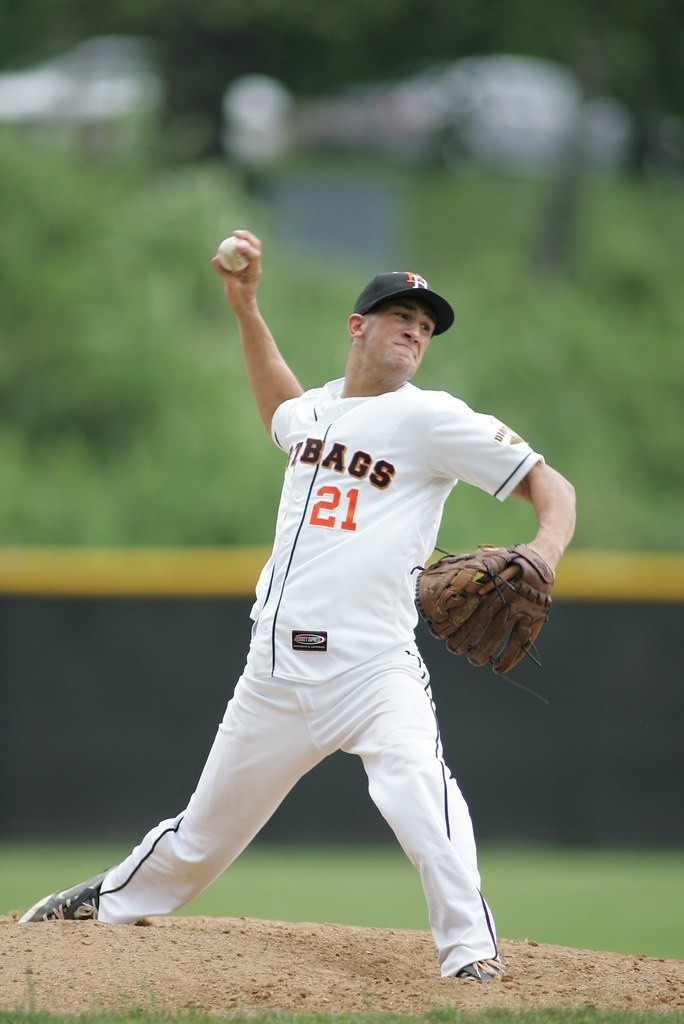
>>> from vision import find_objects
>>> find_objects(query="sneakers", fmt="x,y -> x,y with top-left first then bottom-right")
18,866 -> 121,923
455,961 -> 505,984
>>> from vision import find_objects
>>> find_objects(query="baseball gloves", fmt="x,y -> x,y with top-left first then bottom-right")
415,543 -> 555,675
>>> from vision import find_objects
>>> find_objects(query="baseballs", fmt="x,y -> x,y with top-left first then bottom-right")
217,236 -> 251,272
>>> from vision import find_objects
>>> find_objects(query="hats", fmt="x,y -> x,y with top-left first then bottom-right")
355,272 -> 454,337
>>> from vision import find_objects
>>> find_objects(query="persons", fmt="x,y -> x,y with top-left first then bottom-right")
19,229 -> 575,983
